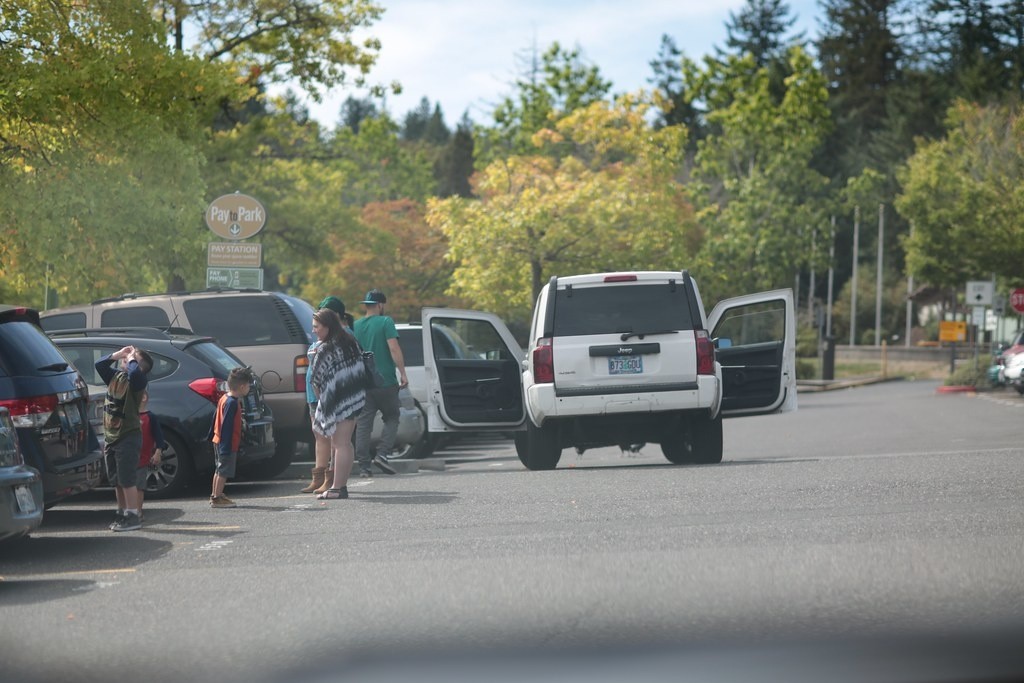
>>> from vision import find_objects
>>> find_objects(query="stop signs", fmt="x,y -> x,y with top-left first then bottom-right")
1010,288 -> 1024,313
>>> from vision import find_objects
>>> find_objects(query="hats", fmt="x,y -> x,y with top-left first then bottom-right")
317,296 -> 345,314
358,291 -> 387,303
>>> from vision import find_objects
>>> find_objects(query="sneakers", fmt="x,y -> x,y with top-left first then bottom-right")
210,495 -> 232,504
211,496 -> 236,507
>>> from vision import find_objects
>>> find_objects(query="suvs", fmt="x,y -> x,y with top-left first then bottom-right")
0,304 -> 108,510
420,270 -> 798,470
387,323 -> 480,460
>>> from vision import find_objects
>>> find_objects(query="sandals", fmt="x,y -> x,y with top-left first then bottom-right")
318,486 -> 347,498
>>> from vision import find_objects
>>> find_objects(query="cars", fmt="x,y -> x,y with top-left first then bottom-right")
0,406 -> 44,540
371,382 -> 425,445
44,326 -> 278,499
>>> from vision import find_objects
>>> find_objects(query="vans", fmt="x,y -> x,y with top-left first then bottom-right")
38,286 -> 318,477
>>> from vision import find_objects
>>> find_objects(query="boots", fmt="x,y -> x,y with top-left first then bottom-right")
301,467 -> 327,492
313,469 -> 335,493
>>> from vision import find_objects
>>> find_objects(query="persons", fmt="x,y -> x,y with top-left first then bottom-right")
309,308 -> 366,500
352,289 -> 409,480
135,390 -> 164,518
206,368 -> 254,508
94,345 -> 153,532
299,296 -> 354,496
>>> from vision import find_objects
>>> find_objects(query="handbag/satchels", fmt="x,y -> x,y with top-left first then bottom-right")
361,350 -> 383,388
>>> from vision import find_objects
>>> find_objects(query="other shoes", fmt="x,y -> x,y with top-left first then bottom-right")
361,468 -> 373,477
108,514 -> 123,528
111,511 -> 142,530
374,454 -> 397,473
